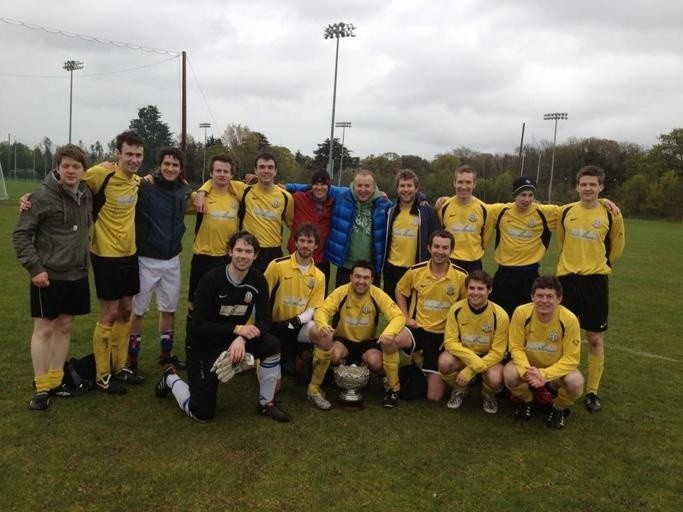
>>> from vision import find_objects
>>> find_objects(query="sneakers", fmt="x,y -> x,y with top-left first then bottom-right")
583,391 -> 603,412
157,353 -> 187,370
305,383 -> 332,412
47,383 -> 75,398
153,362 -> 176,399
446,385 -> 470,409
28,389 -> 52,411
255,398 -> 290,424
93,373 -> 127,395
381,385 -> 402,410
125,360 -> 138,375
512,398 -> 539,420
480,386 -> 500,414
109,366 -> 145,386
542,404 -> 570,430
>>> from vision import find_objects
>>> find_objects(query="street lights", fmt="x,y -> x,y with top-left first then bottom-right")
335,121 -> 351,187
543,113 -> 568,204
198,123 -> 210,185
322,22 -> 357,186
61,60 -> 84,146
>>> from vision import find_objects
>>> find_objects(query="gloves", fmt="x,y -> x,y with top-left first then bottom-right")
210,348 -> 257,385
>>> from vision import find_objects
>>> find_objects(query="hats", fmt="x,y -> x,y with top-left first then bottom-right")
311,168 -> 331,187
511,176 -> 538,199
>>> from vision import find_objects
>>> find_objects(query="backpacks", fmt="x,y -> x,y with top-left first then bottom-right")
60,352 -> 97,393
